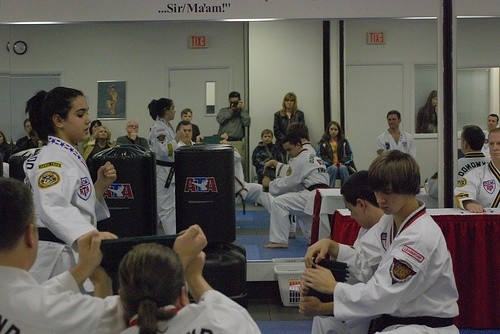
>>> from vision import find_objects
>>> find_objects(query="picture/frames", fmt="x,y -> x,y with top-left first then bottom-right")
97,80 -> 126,120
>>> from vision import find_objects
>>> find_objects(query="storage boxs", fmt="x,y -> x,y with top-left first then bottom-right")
273,258 -> 307,307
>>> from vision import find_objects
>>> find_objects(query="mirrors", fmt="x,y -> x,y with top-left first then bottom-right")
0,15 -> 500,262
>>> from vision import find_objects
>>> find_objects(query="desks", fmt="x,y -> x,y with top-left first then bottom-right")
311,189 -> 437,243
331,208 -> 500,330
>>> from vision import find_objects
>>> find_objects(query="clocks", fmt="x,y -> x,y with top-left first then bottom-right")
13,40 -> 27,55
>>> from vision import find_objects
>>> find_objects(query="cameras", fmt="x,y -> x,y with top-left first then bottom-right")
230,102 -> 238,107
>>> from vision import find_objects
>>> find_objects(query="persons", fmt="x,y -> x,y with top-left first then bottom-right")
119,225 -> 262,334
454,128 -> 500,213
274,91 -> 309,164
24,87 -> 117,295
298,150 -> 459,334
107,83 -> 118,116
316,120 -> 357,188
0,118 -> 44,181
0,176 -> 208,334
252,122 -> 329,249
375,109 -> 416,159
416,114 -> 500,213
216,90 -> 251,183
416,90 -> 438,133
74,120 -> 148,161
147,98 -> 269,235
